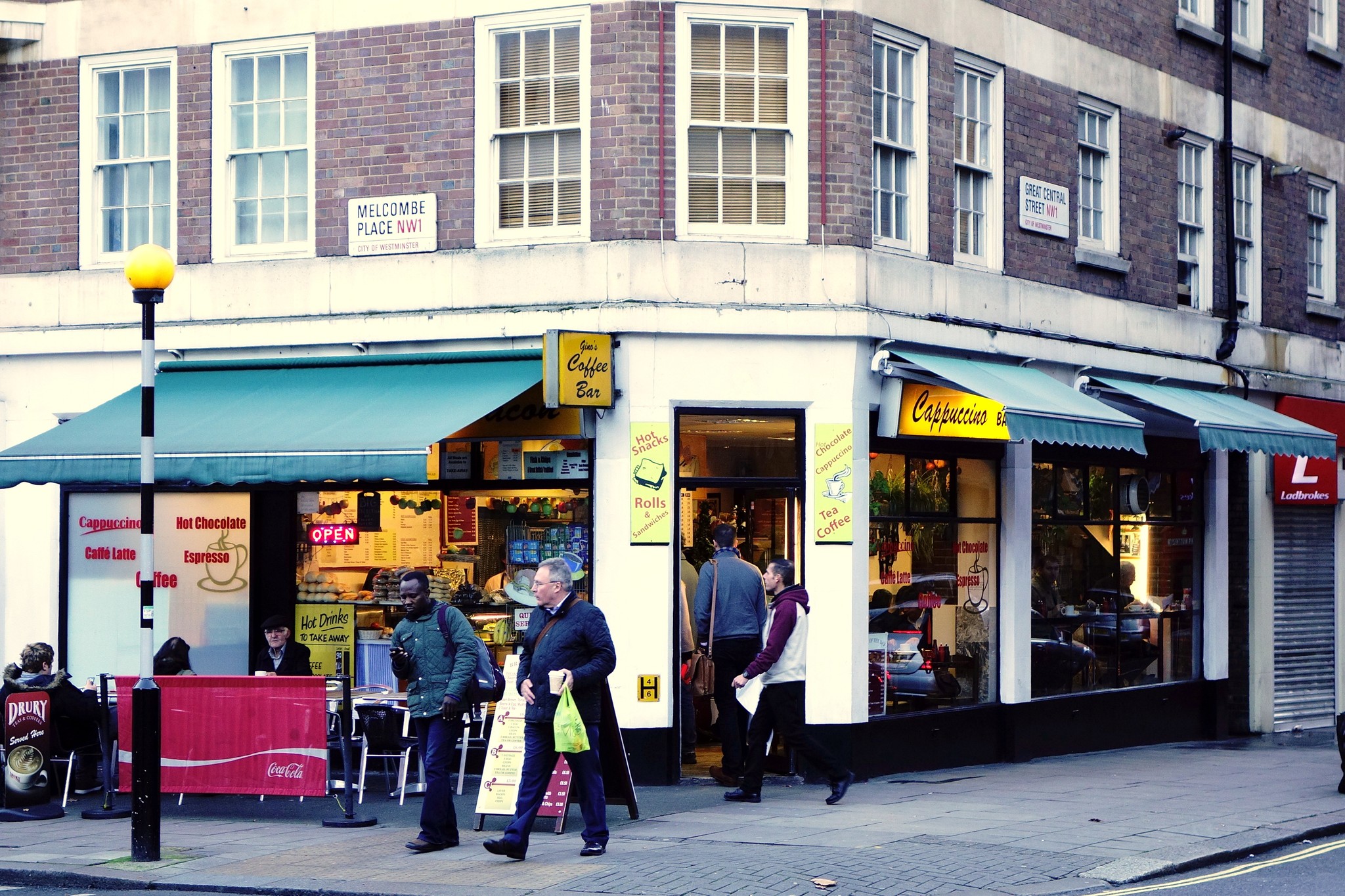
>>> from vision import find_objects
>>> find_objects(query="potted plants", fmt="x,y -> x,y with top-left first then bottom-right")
1033,439 -> 1120,553
869,451 -> 951,564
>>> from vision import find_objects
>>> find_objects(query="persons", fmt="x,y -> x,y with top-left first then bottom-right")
250,615 -> 313,677
152,637 -> 198,676
1032,555 -> 1095,695
679,532 -> 704,765
484,541 -> 521,595
482,558 -> 616,861
867,584 -> 936,659
389,570 -> 483,850
1088,559 -> 1162,688
722,557 -> 854,806
0,642 -> 104,793
693,524 -> 767,789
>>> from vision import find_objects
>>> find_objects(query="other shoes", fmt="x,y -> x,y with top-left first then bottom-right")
682,752 -> 697,764
699,737 -> 722,746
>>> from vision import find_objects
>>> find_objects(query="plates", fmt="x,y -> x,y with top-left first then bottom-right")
1064,614 -> 1081,616
1129,609 -> 1142,613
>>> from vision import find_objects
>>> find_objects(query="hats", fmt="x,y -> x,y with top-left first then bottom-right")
504,569 -> 542,606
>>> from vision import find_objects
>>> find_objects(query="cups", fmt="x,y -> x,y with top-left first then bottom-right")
1128,604 -> 1141,610
1061,606 -> 1074,615
548,671 -> 565,694
255,670 -> 268,677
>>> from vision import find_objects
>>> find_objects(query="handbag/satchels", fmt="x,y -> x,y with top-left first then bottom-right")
689,649 -> 715,697
553,685 -> 590,754
437,605 -> 505,703
709,766 -> 737,787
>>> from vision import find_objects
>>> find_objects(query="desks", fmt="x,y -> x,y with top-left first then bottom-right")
1030,608 -> 1204,698
209,693 -> 261,698
268,690 -> 381,795
364,689 -> 456,799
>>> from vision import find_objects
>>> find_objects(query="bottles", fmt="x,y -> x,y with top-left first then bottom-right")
938,643 -> 944,662
944,644 -> 949,663
932,639 -> 939,663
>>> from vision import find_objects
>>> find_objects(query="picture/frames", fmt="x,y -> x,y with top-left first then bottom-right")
1119,529 -> 1141,559
880,564 -> 893,579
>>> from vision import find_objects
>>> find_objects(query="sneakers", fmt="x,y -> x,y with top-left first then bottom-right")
75,778 -> 104,794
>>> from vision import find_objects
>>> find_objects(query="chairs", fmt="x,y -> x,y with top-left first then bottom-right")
354,703 -> 428,806
170,708 -> 251,806
257,702 -> 349,802
455,701 -> 491,795
319,679 -> 344,732
329,685 -> 399,795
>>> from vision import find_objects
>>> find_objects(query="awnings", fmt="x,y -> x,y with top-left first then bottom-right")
0,349 -> 543,490
1087,375 -> 1339,461
883,351 -> 1148,456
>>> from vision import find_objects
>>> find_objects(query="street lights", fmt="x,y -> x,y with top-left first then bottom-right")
118,240 -> 186,862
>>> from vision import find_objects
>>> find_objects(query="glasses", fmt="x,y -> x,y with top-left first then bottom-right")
533,581 -> 562,587
264,629 -> 285,636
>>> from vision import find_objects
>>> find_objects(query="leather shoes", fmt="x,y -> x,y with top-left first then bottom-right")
723,788 -> 761,802
484,839 -> 525,860
405,839 -> 444,852
447,840 -> 459,847
826,770 -> 855,805
580,841 -> 606,856
